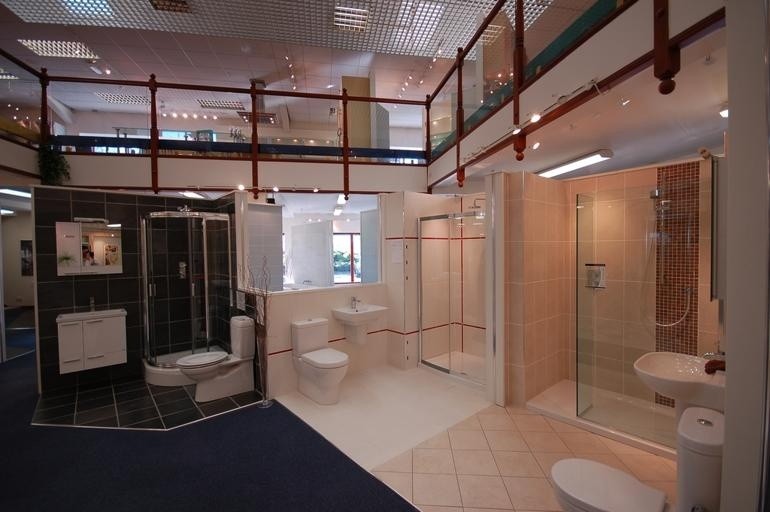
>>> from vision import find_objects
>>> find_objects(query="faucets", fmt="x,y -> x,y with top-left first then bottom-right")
351,296 -> 361,309
89,295 -> 96,311
701,351 -> 726,360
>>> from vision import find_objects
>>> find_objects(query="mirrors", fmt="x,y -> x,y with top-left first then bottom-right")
79,223 -> 123,276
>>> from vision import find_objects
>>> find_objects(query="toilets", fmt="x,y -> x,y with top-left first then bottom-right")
549,407 -> 721,512
176,314 -> 255,403
289,317 -> 349,405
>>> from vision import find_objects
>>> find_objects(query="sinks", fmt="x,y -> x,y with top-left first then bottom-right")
344,307 -> 375,313
633,349 -> 726,412
82,312 -> 105,316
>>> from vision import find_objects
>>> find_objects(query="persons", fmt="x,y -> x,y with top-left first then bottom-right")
84,252 -> 96,267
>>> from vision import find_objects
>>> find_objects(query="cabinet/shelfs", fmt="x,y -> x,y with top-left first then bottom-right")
56,221 -> 81,276
54,308 -> 129,376
